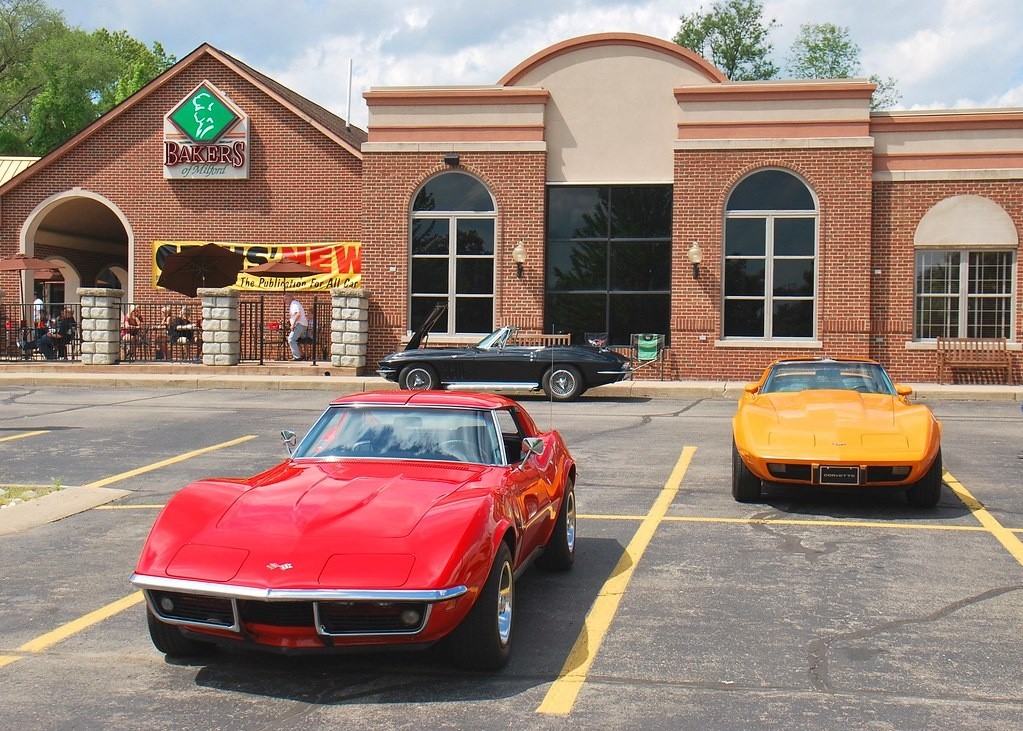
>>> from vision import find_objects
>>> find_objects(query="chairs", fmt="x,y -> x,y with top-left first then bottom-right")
629,333 -> 667,380
58,323 -> 78,359
457,426 -> 493,465
584,333 -> 609,351
781,373 -> 817,390
119,323 -> 327,361
358,422 -> 399,453
837,375 -> 878,392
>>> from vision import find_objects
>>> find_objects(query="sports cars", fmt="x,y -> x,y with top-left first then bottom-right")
130,388 -> 576,669
375,303 -> 636,402
731,356 -> 942,508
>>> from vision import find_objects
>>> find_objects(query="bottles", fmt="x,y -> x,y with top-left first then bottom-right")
282,319 -> 284,329
278,320 -> 282,330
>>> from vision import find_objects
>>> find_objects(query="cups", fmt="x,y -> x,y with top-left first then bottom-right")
39,322 -> 44,327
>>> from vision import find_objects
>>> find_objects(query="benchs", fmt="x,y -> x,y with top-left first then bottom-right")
398,429 -> 524,464
936,335 -> 1014,386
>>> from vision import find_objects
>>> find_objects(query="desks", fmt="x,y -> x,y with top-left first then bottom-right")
135,323 -> 168,361
8,327 -> 49,362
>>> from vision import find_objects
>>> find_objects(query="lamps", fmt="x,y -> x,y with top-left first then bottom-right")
444,154 -> 460,165
512,241 -> 527,278
688,241 -> 703,279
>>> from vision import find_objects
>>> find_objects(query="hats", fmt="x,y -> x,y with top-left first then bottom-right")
162,305 -> 171,314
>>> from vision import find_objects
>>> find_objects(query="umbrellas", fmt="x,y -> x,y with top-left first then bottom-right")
0,254 -> 66,320
241,256 -> 330,361
156,242 -> 247,298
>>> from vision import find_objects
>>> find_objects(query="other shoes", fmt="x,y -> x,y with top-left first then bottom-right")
289,357 -> 302,361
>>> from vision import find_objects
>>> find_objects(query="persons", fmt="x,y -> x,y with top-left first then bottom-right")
16,290 -> 78,362
296,308 -> 321,361
151,307 -> 202,364
120,306 -> 145,360
283,294 -> 309,362
